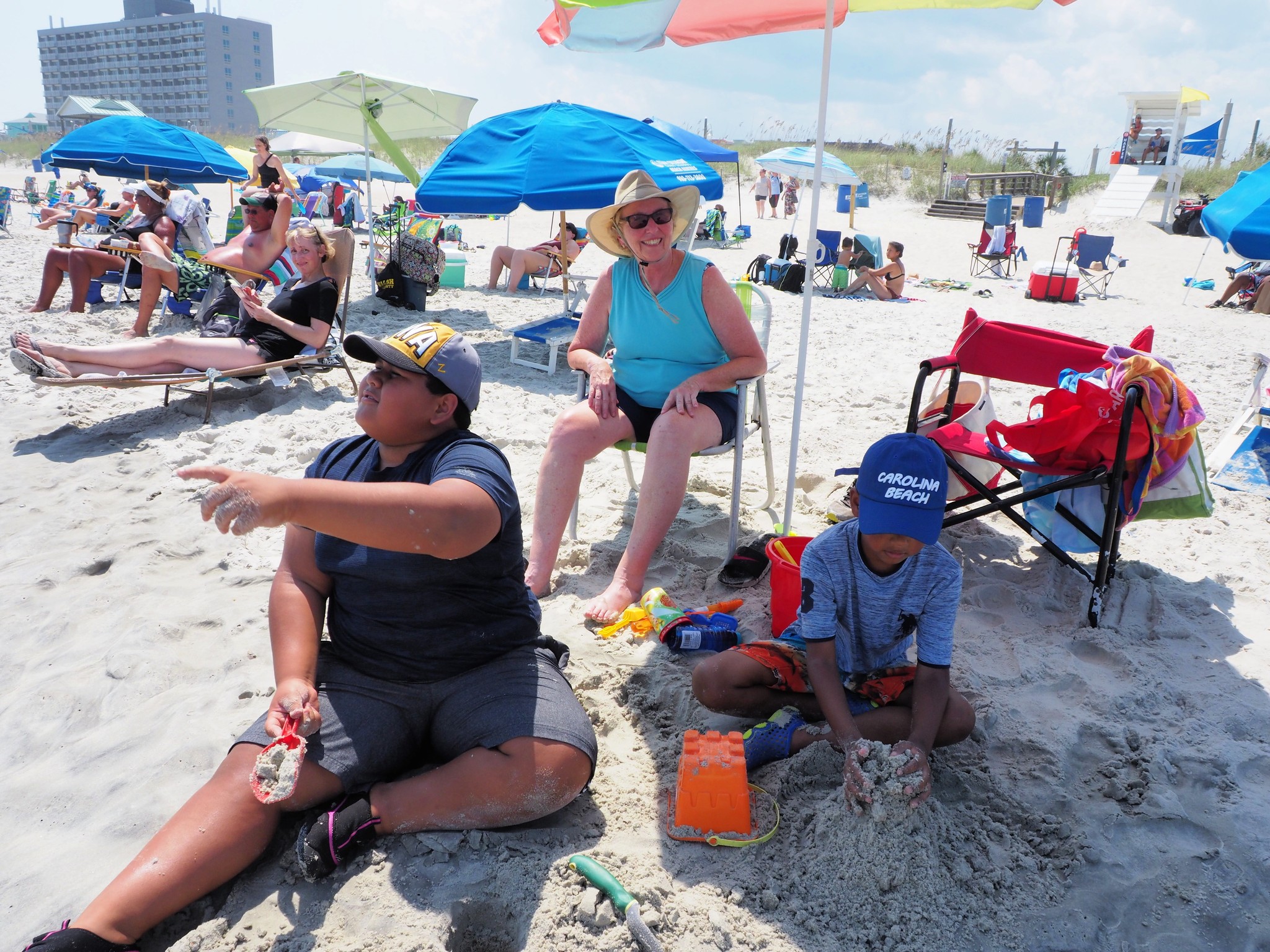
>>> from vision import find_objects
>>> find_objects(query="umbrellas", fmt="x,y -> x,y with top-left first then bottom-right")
415,99 -> 725,313
755,144 -> 863,260
41,116 -> 299,209
242,70 -> 478,295
537,0 -> 1080,539
1200,162 -> 1270,261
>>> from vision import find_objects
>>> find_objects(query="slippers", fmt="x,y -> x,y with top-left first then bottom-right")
718,527 -> 779,594
9,331 -> 73,381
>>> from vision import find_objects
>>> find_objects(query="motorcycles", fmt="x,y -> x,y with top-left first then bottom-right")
1172,193 -> 1217,237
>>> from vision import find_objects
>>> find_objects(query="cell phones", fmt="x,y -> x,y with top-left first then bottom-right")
230,284 -> 251,302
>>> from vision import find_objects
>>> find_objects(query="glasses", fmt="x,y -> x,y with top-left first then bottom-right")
244,208 -> 260,216
85,189 -> 91,192
393,200 -> 398,202
621,207 -> 674,229
713,208 -> 718,210
566,227 -> 571,231
287,223 -> 323,245
133,193 -> 143,199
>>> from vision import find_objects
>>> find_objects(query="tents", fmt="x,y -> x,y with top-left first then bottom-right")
249,132 -> 376,226
640,117 -> 742,230
1182,171 -> 1270,305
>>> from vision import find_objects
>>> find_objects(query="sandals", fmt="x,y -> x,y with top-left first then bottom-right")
844,694 -> 880,717
735,707 -> 802,771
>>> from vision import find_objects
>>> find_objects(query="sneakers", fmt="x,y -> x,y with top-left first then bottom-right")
295,778 -> 382,885
21,919 -> 141,952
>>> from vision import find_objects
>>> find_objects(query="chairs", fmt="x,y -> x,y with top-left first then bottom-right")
1066,226 -> 1129,303
967,219 -> 1019,280
793,228 -> 883,295
1201,349 -> 1270,502
0,175 -> 453,429
902,305 -> 1159,630
501,205 -> 781,573
1224,261 -> 1264,313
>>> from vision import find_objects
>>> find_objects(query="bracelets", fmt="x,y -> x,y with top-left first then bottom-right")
275,194 -> 279,203
263,188 -> 268,193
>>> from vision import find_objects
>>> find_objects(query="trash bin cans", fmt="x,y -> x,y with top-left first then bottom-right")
1023,196 -> 1045,227
984,196 -> 1008,226
855,182 -> 869,208
836,184 -> 851,213
992,195 -> 1012,226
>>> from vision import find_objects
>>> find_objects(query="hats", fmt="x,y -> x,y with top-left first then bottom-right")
84,184 -> 97,191
786,234 -> 798,256
835,434 -> 949,547
129,180 -> 169,206
239,192 -> 278,211
344,321 -> 483,414
585,170 -> 702,258
122,186 -> 136,194
559,221 -> 577,237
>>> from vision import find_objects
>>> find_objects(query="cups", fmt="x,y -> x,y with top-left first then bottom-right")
640,587 -> 693,644
57,219 -> 78,249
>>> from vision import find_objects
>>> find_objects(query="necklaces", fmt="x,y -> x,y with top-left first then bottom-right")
141,213 -> 162,224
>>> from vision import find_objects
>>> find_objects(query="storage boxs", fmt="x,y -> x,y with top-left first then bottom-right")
1025,261 -> 1083,304
761,257 -> 792,286
433,247 -> 469,289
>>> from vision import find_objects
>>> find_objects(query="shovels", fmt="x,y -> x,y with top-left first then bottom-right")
248,711 -> 308,806
568,854 -> 665,952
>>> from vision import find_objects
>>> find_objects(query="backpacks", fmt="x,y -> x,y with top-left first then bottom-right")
773,264 -> 806,294
772,176 -> 784,193
747,253 -> 772,286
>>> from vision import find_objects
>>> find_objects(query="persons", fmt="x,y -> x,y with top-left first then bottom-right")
294,157 -> 300,164
525,169 -> 767,624
35,174 -> 135,233
749,169 -> 800,219
486,222 -> 580,295
372,196 -> 404,227
1220,261 -> 1270,311
27,179 -> 176,313
240,135 -> 301,204
10,223 -> 339,378
123,177 -> 293,340
1129,114 -> 1165,165
20,321 -> 598,952
327,182 -> 341,204
831,237 -> 905,301
691,433 -> 976,815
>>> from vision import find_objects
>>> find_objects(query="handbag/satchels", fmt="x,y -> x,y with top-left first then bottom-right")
791,195 -> 799,204
986,378 -> 1150,477
393,232 -> 445,296
733,225 -> 752,238
374,260 -> 405,305
915,358 -> 1007,507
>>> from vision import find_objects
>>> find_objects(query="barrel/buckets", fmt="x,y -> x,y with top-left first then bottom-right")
1023,196 -> 1045,228
992,194 -> 1012,225
836,184 -> 851,213
855,182 -> 869,208
985,198 -> 1007,226
666,730 -> 780,847
517,274 -> 529,290
765,536 -> 815,638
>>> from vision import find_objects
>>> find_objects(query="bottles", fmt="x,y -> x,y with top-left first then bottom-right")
667,624 -> 742,653
684,599 -> 743,618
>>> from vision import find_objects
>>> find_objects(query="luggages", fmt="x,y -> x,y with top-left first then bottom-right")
1024,237 -> 1079,303
380,203 -> 428,311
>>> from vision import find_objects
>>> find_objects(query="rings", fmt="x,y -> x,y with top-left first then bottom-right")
252,313 -> 254,316
